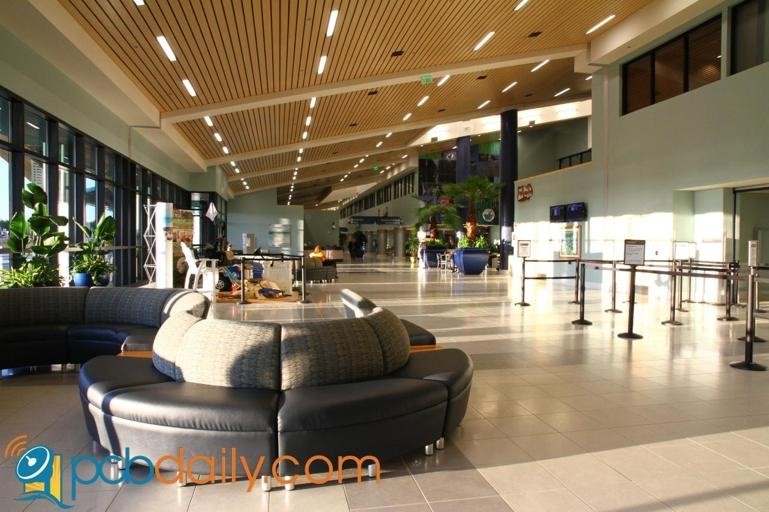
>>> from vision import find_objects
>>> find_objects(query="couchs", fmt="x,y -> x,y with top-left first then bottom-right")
296,256 -> 337,283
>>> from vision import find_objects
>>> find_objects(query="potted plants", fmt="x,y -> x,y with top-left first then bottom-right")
406,234 -> 491,275
0,183 -> 118,286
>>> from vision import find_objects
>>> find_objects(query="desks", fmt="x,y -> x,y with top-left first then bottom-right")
304,250 -> 344,262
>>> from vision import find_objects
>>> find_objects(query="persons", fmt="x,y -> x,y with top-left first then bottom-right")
356,231 -> 368,262
308,245 -> 332,267
349,235 -> 356,259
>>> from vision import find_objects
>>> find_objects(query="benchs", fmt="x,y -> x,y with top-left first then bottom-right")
0,286 -> 474,492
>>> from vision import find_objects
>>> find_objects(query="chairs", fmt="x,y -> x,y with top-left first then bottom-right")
181,242 -> 219,302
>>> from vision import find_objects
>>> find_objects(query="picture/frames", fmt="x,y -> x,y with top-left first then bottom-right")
559,225 -> 582,260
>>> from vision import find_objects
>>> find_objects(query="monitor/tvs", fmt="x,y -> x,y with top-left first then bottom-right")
268,246 -> 282,254
550,204 -> 566,223
566,202 -> 587,222
253,246 -> 262,253
